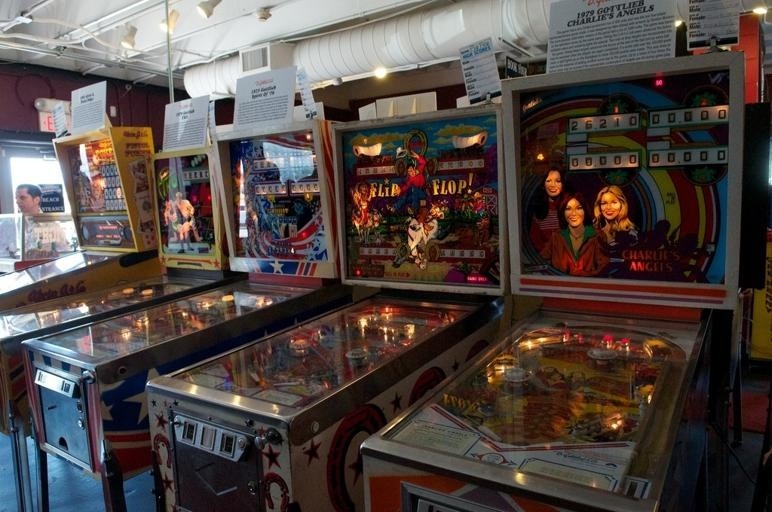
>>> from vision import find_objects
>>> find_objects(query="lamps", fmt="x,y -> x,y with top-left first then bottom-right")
122,0 -> 224,48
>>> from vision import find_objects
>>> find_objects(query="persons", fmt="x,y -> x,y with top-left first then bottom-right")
387,150 -> 427,213
15,184 -> 70,252
530,170 -> 638,275
164,192 -> 203,242
